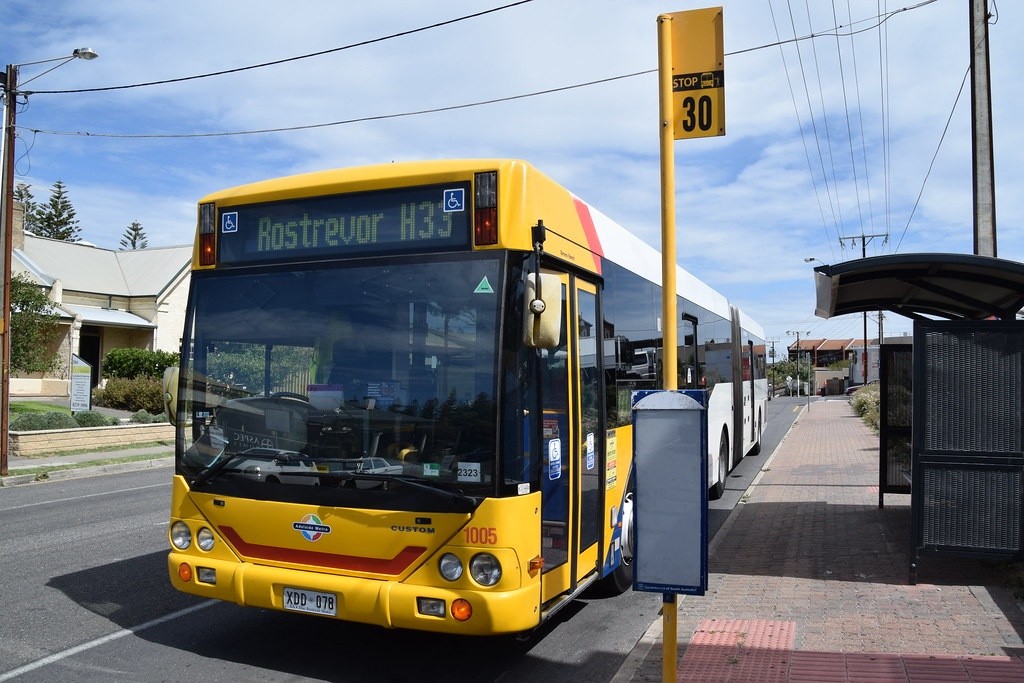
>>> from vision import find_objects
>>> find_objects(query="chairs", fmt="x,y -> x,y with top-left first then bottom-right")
397,369 -> 438,410
328,339 -> 391,408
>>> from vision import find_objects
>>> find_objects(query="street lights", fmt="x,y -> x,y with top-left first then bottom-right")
2,48 -> 100,479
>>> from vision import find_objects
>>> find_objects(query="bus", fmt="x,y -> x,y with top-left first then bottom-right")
162,158 -> 766,635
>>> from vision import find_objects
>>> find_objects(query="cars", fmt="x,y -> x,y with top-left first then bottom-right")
845,379 -> 879,397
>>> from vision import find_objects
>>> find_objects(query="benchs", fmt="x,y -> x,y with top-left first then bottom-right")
901,470 -> 912,484
